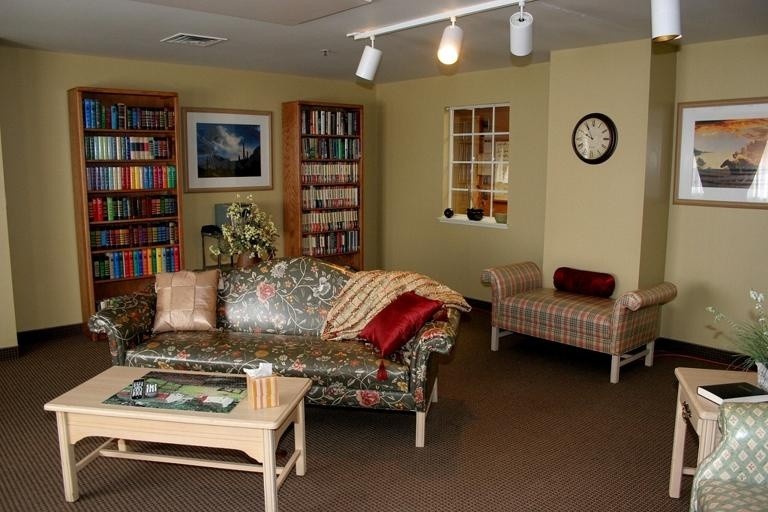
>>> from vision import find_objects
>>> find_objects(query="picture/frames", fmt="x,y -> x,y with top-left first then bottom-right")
671,96 -> 768,211
180,106 -> 275,196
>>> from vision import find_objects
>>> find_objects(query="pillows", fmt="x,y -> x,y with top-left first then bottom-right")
357,289 -> 441,357
552,265 -> 616,300
148,266 -> 222,335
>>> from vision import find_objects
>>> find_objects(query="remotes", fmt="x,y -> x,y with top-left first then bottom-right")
132,379 -> 143,398
145,383 -> 158,397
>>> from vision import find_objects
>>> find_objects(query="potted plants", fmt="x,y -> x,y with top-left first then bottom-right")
465,184 -> 484,221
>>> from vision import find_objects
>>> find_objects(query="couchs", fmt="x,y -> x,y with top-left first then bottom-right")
86,254 -> 475,451
690,400 -> 768,511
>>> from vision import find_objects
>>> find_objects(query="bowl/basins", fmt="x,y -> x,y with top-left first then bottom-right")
494,213 -> 507,224
466,208 -> 484,221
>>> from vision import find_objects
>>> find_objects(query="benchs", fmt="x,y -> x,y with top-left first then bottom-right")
479,260 -> 678,384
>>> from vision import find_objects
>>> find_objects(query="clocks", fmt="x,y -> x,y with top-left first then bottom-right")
571,110 -> 618,165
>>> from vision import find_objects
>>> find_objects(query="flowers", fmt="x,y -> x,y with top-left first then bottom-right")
212,193 -> 278,259
706,284 -> 768,379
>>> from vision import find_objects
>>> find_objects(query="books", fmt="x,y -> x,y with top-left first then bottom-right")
301,105 -> 359,259
696,382 -> 766,410
83,95 -> 179,285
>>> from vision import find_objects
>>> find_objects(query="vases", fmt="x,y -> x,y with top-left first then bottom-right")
494,211 -> 507,224
753,360 -> 768,389
237,250 -> 255,270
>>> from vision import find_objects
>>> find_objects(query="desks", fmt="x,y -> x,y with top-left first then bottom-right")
666,366 -> 767,500
41,363 -> 315,511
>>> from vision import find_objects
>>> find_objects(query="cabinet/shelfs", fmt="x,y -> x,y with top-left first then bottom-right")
280,99 -> 367,276
66,85 -> 186,342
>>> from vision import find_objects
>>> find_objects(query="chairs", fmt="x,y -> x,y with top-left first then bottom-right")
214,202 -> 246,268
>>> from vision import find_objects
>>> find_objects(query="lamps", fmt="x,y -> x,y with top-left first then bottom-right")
351,1 -> 535,85
646,0 -> 682,45
